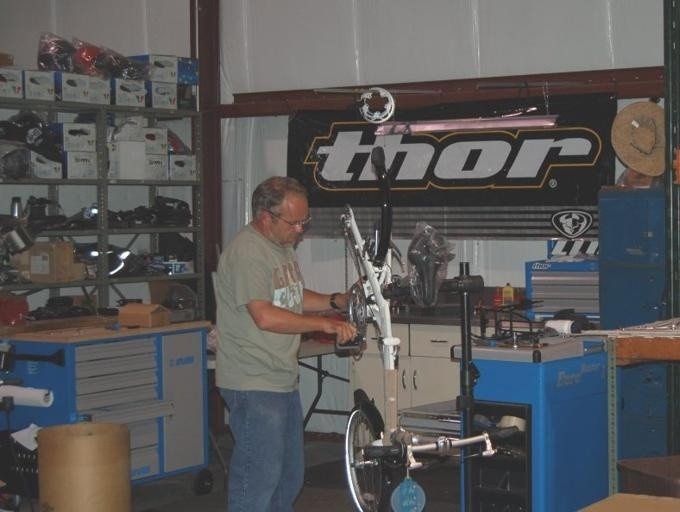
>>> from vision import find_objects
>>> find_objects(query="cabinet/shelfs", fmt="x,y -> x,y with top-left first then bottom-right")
0,96 -> 206,321
347,312 -> 544,421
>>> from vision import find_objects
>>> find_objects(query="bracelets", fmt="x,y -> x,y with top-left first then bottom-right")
329,292 -> 342,310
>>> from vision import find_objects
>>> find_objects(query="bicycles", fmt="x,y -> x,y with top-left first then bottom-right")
338,146 -> 522,512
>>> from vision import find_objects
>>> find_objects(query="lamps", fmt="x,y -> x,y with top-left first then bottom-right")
372,82 -> 559,137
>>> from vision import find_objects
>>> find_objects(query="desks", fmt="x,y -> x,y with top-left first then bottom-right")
197,335 -> 350,482
601,314 -> 679,494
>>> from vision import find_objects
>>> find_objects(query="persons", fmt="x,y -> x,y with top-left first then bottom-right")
214,175 -> 358,512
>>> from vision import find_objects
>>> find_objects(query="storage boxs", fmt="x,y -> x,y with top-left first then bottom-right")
118,301 -> 172,328
51,240 -> 73,282
0,52 -> 197,184
11,241 -> 57,283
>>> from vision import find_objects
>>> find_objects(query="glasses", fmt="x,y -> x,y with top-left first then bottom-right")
261,207 -> 312,227
